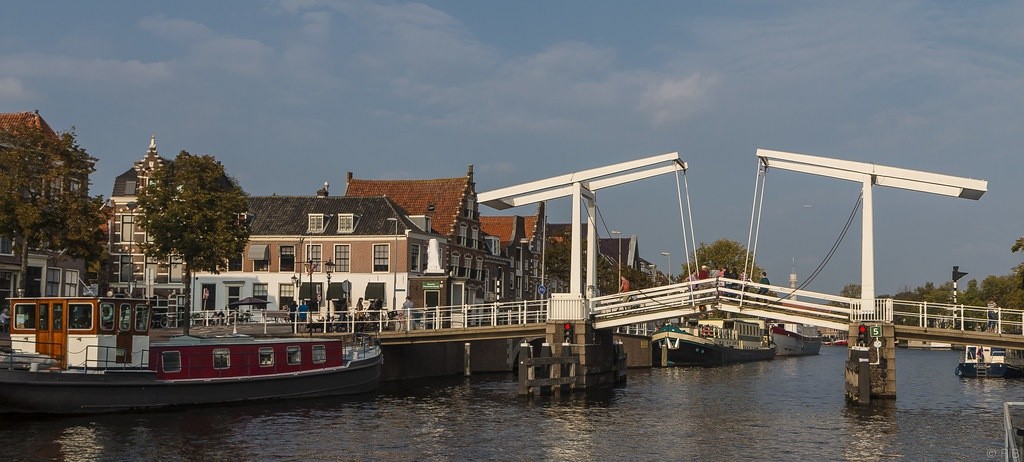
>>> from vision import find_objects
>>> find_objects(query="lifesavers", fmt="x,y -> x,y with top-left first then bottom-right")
955,367 -> 960,375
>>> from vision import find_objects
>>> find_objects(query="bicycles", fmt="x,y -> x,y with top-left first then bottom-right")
159,307 -> 255,329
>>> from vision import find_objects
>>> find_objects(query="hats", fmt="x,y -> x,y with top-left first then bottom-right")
702,265 -> 709,269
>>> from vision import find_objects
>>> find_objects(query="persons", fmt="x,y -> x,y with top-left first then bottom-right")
298,301 -> 309,333
217,309 -> 225,324
364,298 -> 382,331
356,297 -> 365,332
738,268 -> 749,305
699,265 -> 713,302
616,275 -> 632,315
0,309 -> 11,332
290,301 -> 299,333
685,269 -> 697,307
756,272 -> 770,310
977,349 -> 981,361
333,300 -> 351,334
718,266 -> 739,302
402,297 -> 414,331
209,311 -> 218,325
987,295 -> 999,333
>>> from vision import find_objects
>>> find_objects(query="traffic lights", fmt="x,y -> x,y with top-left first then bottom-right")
563,322 -> 573,344
858,324 -> 868,344
952,270 -> 969,281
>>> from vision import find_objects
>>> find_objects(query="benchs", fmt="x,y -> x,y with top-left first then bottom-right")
261,309 -> 291,324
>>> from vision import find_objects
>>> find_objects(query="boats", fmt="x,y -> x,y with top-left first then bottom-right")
768,323 -> 823,358
651,316 -> 779,369
0,293 -> 387,420
955,342 -> 1009,379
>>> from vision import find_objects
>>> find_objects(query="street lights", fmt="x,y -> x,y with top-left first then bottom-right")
324,258 -> 335,321
661,252 -> 671,295
386,217 -> 398,309
290,274 -> 298,332
612,230 -> 622,297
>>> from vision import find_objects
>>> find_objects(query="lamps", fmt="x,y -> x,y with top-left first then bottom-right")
292,274 -> 298,288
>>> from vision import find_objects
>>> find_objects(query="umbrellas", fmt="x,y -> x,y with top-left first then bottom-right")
228,296 -> 274,309
